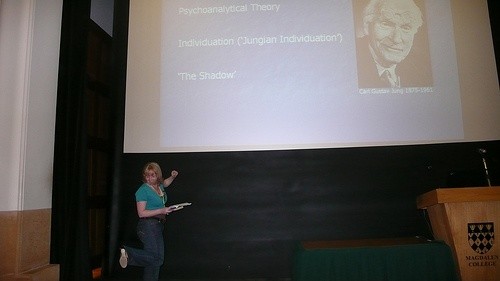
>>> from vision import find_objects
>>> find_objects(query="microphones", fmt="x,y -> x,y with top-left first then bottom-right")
475,147 -> 488,156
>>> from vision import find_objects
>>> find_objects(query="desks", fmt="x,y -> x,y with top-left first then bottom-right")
291,236 -> 459,281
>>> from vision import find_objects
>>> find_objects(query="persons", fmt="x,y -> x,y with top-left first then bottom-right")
119,163 -> 179,281
354,0 -> 433,88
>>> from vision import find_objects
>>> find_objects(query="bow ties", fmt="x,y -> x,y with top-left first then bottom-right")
377,64 -> 395,77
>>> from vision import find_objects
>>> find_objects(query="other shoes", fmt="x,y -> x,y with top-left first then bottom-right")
120,249 -> 128,268
